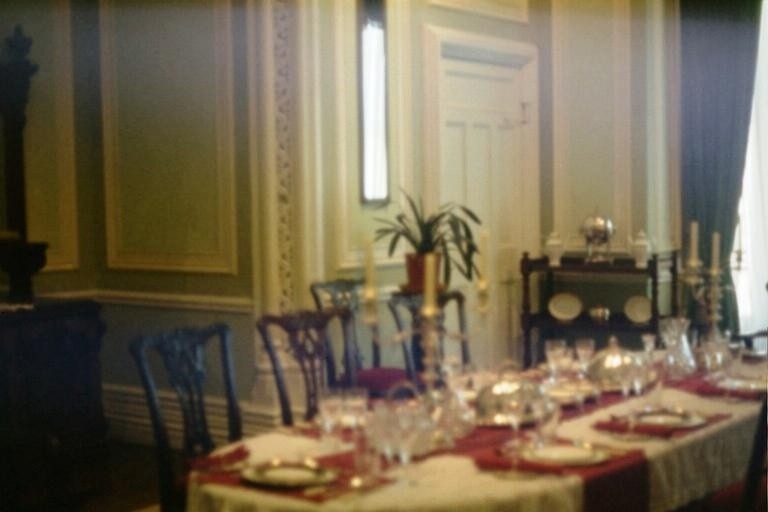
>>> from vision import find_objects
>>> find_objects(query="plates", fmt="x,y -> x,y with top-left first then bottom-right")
241,458 -> 337,490
709,373 -> 766,392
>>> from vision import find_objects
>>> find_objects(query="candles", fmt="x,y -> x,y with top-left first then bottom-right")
689,220 -> 743,271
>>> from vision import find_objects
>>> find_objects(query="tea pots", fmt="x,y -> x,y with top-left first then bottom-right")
626,227 -> 654,269
535,230 -> 573,269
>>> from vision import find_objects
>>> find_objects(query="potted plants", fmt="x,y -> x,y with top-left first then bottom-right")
371,186 -> 482,295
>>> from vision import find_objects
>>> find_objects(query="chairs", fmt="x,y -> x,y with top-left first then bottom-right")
256,307 -> 359,428
309,278 -> 443,399
130,320 -> 244,512
387,290 -> 470,392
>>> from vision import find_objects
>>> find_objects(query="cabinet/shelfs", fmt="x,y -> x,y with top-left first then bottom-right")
521,248 -> 680,367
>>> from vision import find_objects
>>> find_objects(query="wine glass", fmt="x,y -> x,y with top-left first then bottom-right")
313,316 -> 733,492
